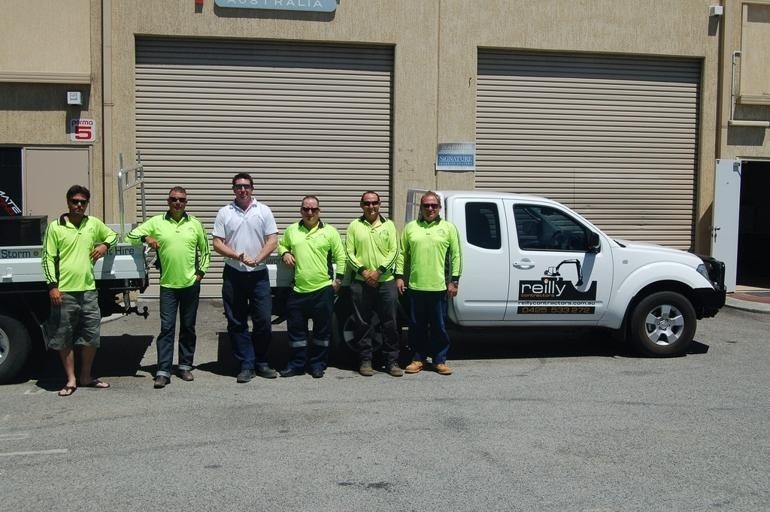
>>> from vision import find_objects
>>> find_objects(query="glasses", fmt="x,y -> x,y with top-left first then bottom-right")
235,184 -> 249,189
171,197 -> 186,202
364,201 -> 379,205
303,207 -> 319,211
71,199 -> 87,204
423,204 -> 439,208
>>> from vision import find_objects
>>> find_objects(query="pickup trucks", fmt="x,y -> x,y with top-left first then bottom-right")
0,152 -> 149,382
266,190 -> 726,357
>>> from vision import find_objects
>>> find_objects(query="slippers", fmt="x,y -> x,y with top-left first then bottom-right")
59,383 -> 76,396
79,379 -> 110,388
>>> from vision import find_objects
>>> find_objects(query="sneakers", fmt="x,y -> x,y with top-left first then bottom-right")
406,361 -> 423,373
360,360 -> 374,375
387,361 -> 403,376
154,376 -> 170,388
280,367 -> 304,376
257,362 -> 277,378
238,368 -> 256,382
177,370 -> 194,381
432,363 -> 454,374
311,367 -> 323,377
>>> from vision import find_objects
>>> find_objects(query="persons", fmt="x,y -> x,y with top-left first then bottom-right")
277,195 -> 347,378
211,172 -> 279,382
41,185 -> 120,396
123,186 -> 211,388
392,190 -> 463,374
344,190 -> 404,376
